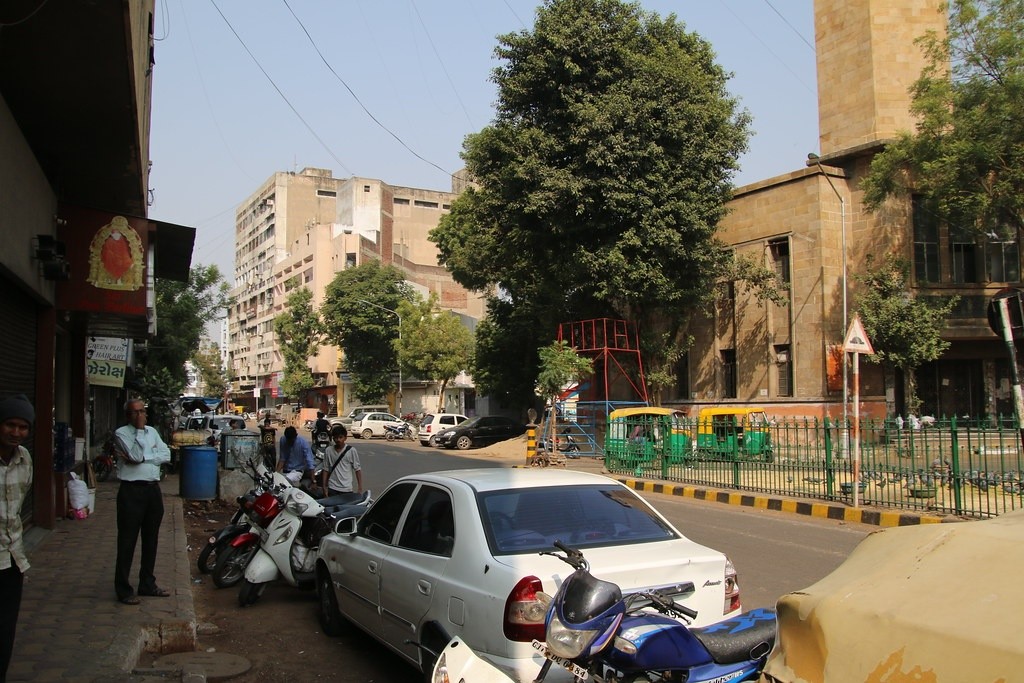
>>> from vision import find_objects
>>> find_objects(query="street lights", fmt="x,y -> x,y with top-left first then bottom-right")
358,299 -> 403,420
223,340 -> 259,420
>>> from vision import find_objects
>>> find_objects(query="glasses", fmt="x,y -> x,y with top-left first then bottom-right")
129,409 -> 147,415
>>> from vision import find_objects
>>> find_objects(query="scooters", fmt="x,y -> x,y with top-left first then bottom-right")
92,425 -> 122,483
197,456 -> 376,607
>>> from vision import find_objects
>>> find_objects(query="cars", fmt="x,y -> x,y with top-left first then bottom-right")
417,412 -> 470,447
312,467 -> 744,683
178,414 -> 249,454
434,414 -> 528,451
350,410 -> 416,440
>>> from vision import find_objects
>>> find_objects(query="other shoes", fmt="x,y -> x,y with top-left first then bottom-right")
121,595 -> 141,604
137,585 -> 171,598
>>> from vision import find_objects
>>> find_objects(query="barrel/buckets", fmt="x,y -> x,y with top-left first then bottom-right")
178,446 -> 217,501
74,438 -> 85,461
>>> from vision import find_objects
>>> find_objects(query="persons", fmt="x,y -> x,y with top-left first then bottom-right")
0,392 -> 35,682
113,399 -> 171,605
630,419 -> 656,443
323,426 -> 363,497
260,419 -> 278,467
949,394 -> 984,426
312,412 -> 332,444
276,427 -> 318,491
220,420 -> 238,454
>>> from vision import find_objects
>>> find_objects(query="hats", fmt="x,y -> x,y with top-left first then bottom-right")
0,393 -> 35,425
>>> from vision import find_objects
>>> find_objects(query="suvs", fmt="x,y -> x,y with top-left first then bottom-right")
323,404 -> 389,435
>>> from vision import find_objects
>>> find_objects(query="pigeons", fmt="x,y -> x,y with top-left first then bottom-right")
788,446 -> 1024,496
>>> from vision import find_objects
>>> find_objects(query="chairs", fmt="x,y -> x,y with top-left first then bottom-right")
428,500 -> 455,555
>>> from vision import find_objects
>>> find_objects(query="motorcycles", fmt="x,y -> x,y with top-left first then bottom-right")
602,406 -> 700,472
695,406 -> 774,464
402,538 -> 776,683
522,419 -> 581,460
383,422 -> 416,442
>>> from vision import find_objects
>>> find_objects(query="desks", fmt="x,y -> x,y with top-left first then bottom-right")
55,458 -> 85,520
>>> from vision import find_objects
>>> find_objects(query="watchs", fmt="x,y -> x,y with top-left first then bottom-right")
313,479 -> 317,483
